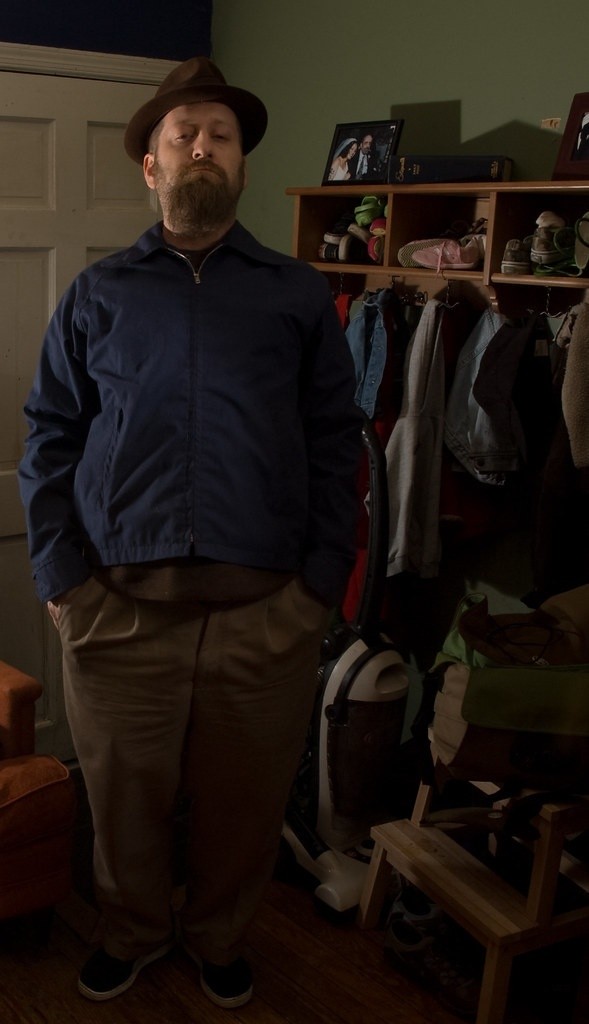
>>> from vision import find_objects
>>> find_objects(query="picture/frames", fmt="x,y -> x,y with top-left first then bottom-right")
321,118 -> 405,186
551,92 -> 589,181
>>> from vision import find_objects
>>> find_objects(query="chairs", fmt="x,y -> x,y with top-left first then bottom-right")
351,765 -> 589,1024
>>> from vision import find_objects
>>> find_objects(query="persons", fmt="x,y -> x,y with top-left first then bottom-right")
18,57 -> 357,1003
328,133 -> 382,181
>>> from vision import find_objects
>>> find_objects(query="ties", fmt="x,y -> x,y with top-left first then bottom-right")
355,156 -> 366,181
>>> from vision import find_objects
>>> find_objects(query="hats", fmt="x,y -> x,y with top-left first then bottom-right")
123,57 -> 268,166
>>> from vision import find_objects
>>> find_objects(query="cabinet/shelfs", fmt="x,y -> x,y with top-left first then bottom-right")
284,177 -> 589,306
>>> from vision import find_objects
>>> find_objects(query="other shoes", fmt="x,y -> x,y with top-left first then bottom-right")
500,211 -> 589,276
181,937 -> 253,1008
397,217 -> 488,280
317,195 -> 388,266
78,939 -> 169,1001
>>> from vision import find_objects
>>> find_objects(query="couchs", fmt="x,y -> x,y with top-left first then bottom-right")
0,658 -> 82,939
